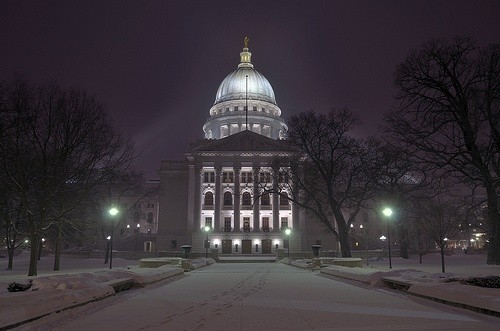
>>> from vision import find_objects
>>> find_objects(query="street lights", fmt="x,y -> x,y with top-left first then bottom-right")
383,207 -> 392,269
109,207 -> 119,270
